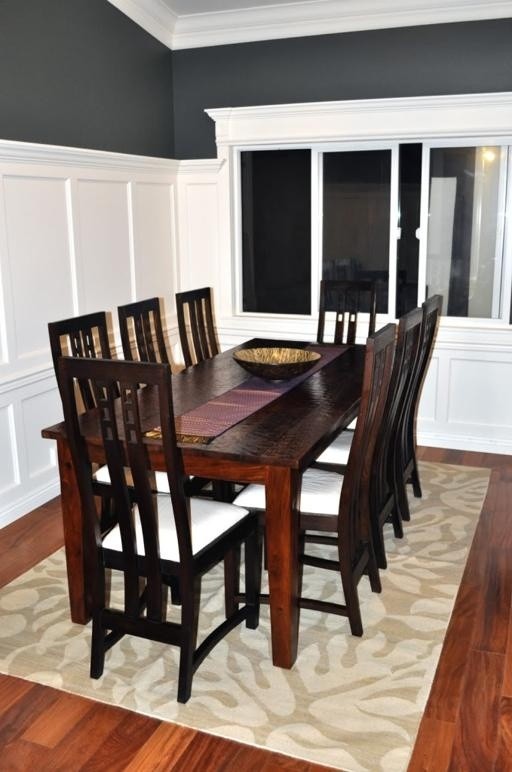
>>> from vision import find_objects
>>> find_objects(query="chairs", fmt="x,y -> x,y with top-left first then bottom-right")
114,292 -> 245,503
51,349 -> 263,706
163,286 -> 221,367
345,292 -> 448,518
315,305 -> 424,568
44,304 -> 238,610
228,323 -> 398,636
311,272 -> 378,353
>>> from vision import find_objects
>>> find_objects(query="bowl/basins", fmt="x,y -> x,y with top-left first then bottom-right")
232,347 -> 323,383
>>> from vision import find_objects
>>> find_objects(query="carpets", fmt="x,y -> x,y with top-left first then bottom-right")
1,455 -> 494,772
